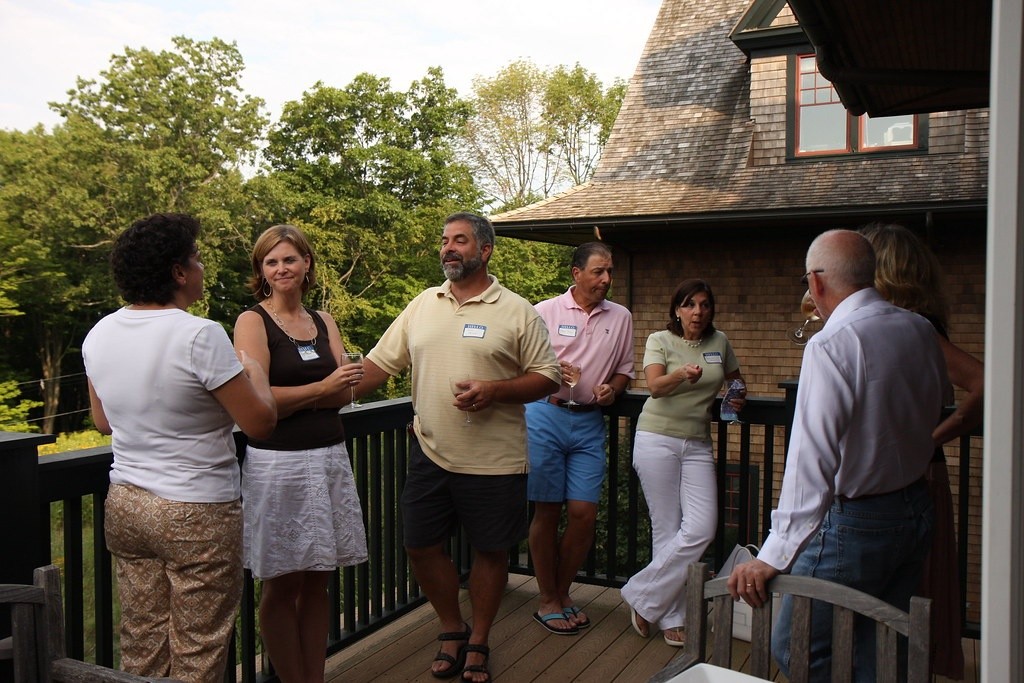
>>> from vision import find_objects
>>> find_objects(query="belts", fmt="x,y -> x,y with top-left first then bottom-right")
544,396 -> 603,413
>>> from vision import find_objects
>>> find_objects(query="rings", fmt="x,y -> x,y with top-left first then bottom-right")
746,583 -> 754,587
473,404 -> 476,411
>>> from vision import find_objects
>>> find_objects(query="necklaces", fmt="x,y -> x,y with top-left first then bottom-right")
266,297 -> 316,350
679,334 -> 704,348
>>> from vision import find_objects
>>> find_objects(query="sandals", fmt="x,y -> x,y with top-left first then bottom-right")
431,623 -> 472,678
462,639 -> 491,683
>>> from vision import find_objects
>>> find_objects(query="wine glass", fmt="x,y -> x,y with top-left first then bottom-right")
786,289 -> 821,346
449,372 -> 478,426
563,363 -> 581,406
340,352 -> 363,409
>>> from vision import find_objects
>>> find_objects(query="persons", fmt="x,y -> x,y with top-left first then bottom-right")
232,225 -> 364,682
521,242 -> 635,635
855,221 -> 985,683
725,228 -> 948,683
83,211 -> 277,683
621,280 -> 747,651
352,212 -> 561,683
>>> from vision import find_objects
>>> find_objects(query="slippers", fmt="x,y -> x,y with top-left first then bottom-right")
564,605 -> 590,629
532,606 -> 580,635
664,625 -> 686,647
621,590 -> 650,639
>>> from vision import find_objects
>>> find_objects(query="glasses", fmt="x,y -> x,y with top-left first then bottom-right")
801,269 -> 825,284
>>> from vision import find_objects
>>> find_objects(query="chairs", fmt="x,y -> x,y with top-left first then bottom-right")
647,560 -> 935,683
